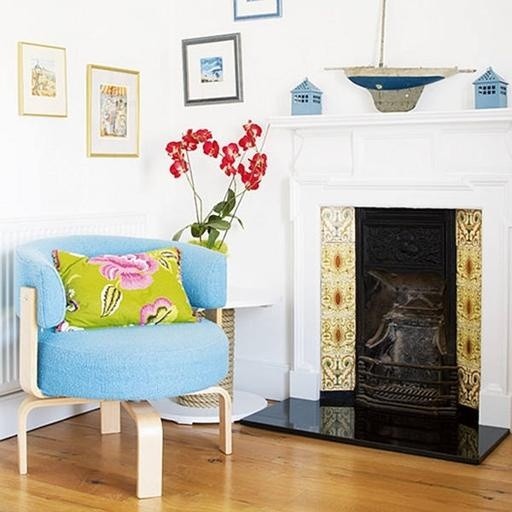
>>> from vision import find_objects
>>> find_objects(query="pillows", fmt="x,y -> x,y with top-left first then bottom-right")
50,249 -> 202,332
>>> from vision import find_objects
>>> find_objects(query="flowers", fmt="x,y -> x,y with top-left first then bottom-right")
167,119 -> 273,249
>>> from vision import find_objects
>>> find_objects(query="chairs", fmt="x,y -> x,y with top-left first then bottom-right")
10,236 -> 231,499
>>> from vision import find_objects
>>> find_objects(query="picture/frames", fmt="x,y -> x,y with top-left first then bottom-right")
86,63 -> 140,158
182,32 -> 244,107
18,42 -> 69,118
234,0 -> 279,20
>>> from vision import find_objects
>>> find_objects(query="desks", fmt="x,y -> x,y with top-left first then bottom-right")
147,299 -> 276,424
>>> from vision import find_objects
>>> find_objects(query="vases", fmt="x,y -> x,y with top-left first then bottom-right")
188,240 -> 228,255
